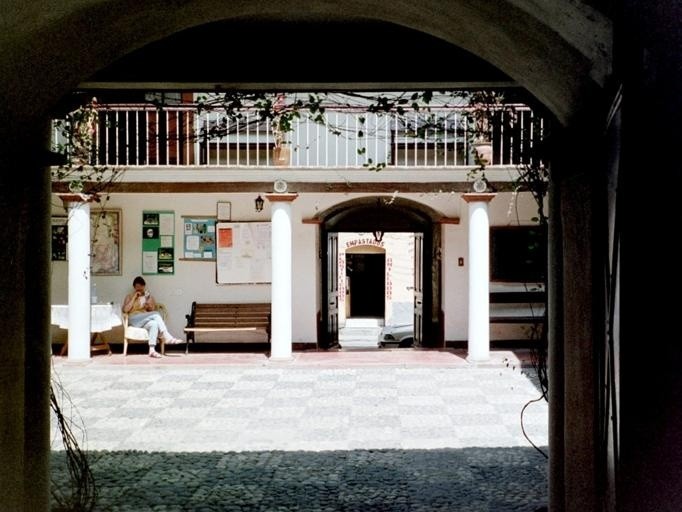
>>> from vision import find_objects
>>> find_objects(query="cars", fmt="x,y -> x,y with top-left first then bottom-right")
377,323 -> 414,348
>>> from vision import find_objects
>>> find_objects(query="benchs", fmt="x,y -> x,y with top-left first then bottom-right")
183,300 -> 271,354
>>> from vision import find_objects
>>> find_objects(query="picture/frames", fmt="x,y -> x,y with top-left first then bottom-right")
90,207 -> 122,276
51,214 -> 68,261
217,201 -> 231,222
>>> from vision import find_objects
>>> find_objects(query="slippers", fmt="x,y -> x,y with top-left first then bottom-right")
150,351 -> 162,358
169,338 -> 182,345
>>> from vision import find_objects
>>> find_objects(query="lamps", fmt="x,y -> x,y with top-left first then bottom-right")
255,196 -> 264,213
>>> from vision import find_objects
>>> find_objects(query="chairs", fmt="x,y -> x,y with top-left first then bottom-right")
120,302 -> 167,355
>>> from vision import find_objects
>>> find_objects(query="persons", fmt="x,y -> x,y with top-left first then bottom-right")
122,277 -> 183,358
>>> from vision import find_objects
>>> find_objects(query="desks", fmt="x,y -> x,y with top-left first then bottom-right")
51,304 -> 122,356
